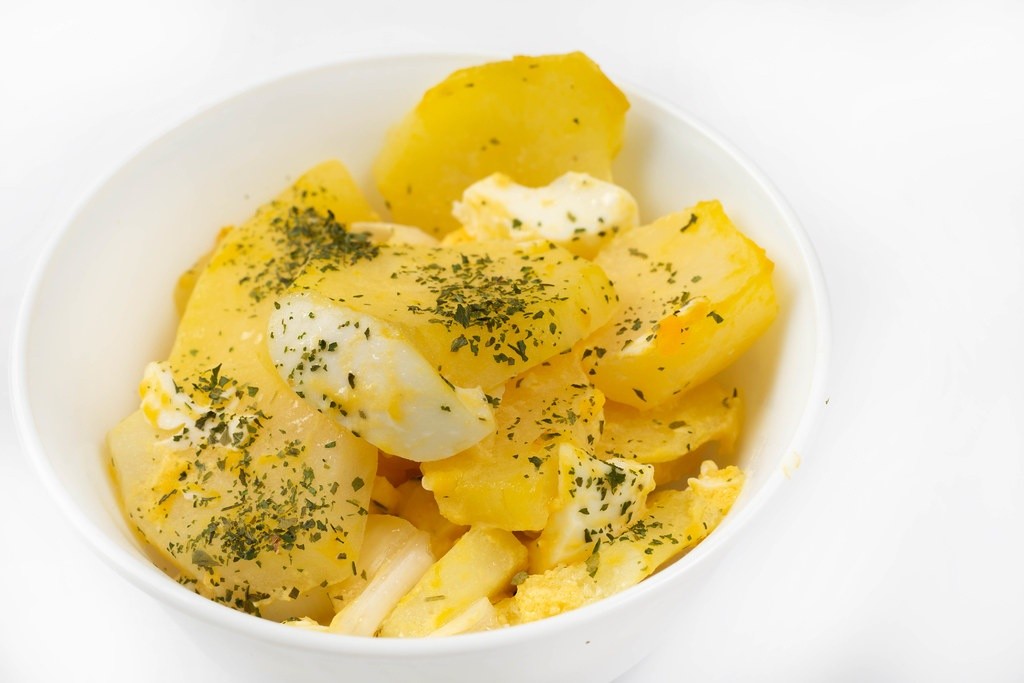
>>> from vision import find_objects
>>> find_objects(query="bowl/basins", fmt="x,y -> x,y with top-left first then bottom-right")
11,47 -> 825,683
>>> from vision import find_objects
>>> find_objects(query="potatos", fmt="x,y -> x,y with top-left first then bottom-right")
111,52 -> 779,639
